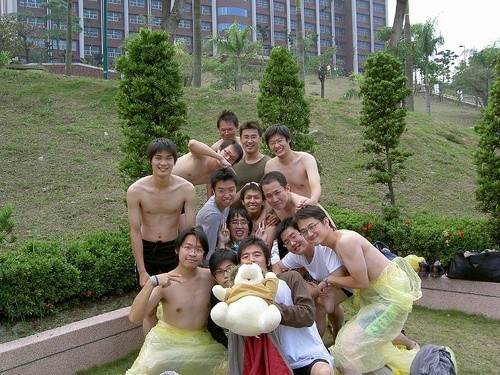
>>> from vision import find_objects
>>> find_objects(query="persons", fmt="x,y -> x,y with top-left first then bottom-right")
126,110 -> 422,375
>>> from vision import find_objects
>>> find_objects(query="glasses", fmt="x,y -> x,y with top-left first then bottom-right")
282,233 -> 301,247
215,268 -> 230,275
299,220 -> 321,237
223,148 -> 236,163
229,220 -> 249,226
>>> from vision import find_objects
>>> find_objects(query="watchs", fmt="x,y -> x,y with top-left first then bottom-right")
323,276 -> 329,285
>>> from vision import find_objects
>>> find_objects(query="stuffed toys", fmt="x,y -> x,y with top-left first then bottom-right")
210,260 -> 281,336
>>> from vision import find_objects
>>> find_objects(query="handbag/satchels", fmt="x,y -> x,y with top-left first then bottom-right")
469,252 -> 500,281
448,248 -> 478,279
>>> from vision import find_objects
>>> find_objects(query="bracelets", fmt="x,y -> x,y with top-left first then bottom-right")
149,275 -> 159,288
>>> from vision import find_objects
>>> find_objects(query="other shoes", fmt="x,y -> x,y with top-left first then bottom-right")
430,264 -> 447,277
419,262 -> 431,277
